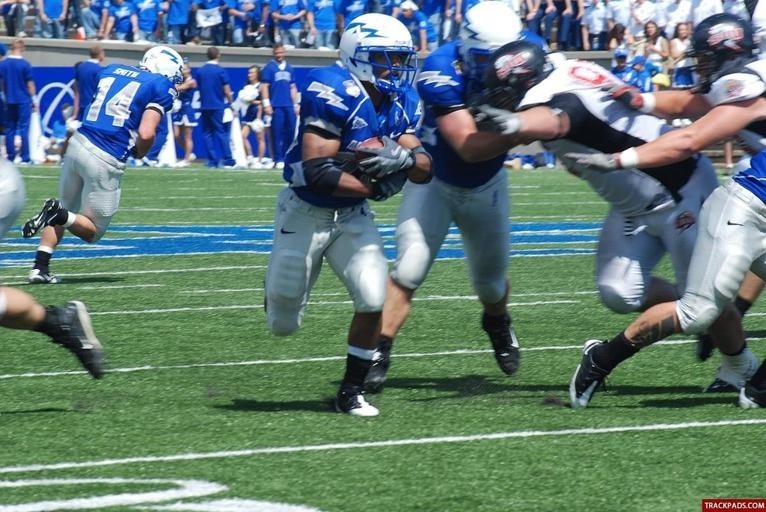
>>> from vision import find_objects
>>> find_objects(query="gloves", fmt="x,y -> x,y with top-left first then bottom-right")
562,146 -> 640,174
600,80 -> 656,115
470,103 -> 524,138
351,134 -> 416,180
359,167 -> 409,203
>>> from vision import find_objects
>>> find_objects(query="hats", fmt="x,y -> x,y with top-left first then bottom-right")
612,47 -> 647,67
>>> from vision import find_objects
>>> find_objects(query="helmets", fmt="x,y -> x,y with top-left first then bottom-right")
138,46 -> 184,87
683,13 -> 760,96
485,41 -> 547,114
459,2 -> 524,82
338,11 -> 419,98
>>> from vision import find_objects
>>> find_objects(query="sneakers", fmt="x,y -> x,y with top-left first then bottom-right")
569,339 -> 612,409
29,267 -> 63,285
131,154 -> 196,168
20,197 -> 63,239
486,322 -> 522,376
209,156 -> 287,170
333,387 -> 381,419
696,337 -> 761,410
363,349 -> 389,394
63,299 -> 106,379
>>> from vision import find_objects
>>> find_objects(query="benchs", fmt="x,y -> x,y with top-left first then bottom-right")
1,1 -> 82,41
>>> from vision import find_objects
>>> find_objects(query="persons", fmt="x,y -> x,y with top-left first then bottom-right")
566,143 -> 765,412
465,39 -> 765,393
262,10 -> 435,417
360,0 -> 564,398
0,156 -> 109,381
561,13 -> 765,174
20,43 -> 186,285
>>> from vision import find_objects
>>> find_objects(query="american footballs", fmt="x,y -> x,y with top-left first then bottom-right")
356,136 -> 383,152
